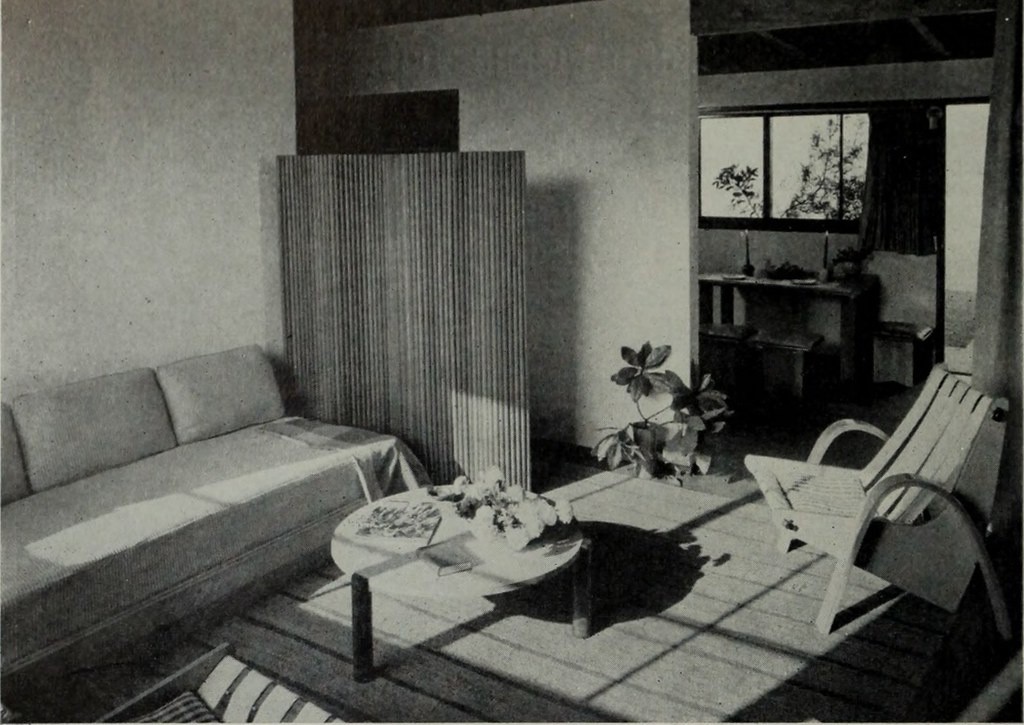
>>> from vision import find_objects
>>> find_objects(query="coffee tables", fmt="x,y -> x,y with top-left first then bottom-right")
328,485 -> 596,682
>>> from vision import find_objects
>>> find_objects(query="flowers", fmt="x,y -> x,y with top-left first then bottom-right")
446,464 -> 574,550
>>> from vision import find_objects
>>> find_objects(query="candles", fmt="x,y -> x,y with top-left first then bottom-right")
822,230 -> 830,269
744,229 -> 750,265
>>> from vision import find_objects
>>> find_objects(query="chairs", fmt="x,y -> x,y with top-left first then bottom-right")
742,362 -> 1018,646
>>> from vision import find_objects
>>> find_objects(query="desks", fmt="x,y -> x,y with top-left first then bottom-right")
697,271 -> 882,384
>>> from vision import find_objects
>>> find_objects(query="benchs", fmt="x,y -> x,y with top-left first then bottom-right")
699,323 -> 824,397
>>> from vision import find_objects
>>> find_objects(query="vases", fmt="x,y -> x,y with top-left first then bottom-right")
462,519 -> 520,546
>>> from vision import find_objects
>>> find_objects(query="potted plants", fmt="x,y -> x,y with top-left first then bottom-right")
832,246 -> 872,275
590,340 -> 730,486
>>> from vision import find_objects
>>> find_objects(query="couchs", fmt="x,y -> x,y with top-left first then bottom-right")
2,344 -> 431,695
